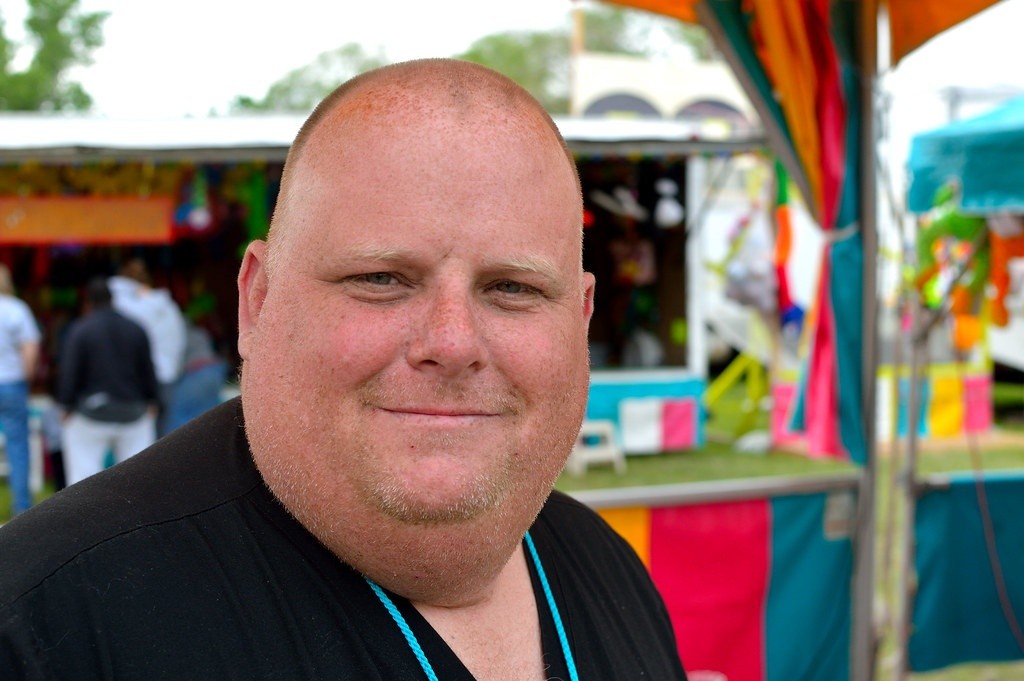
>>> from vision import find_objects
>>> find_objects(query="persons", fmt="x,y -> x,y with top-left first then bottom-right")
0,59 -> 688,681
0,253 -> 189,516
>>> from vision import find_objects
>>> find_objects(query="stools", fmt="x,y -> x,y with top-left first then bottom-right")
565,421 -> 628,477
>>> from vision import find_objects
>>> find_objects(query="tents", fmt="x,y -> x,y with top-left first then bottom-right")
908,96 -> 1024,218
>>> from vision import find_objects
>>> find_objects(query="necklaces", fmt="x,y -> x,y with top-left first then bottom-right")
365,529 -> 579,681
